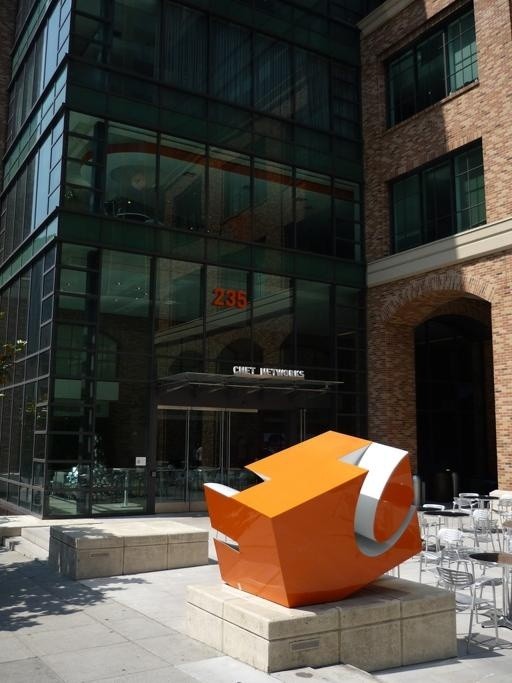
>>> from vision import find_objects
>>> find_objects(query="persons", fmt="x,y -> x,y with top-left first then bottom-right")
193,442 -> 203,467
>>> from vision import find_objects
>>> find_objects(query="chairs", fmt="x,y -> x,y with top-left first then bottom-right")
412,490 -> 511,656
50,466 -> 258,505
